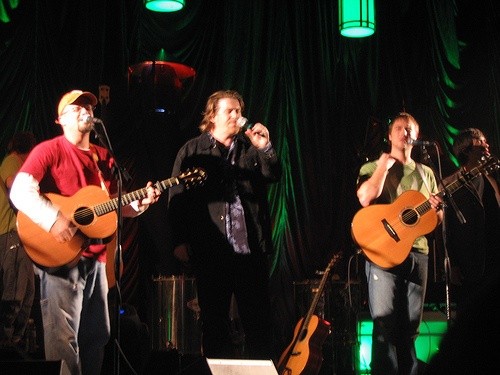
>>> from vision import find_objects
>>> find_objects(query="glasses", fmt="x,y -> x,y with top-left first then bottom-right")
59,104 -> 97,116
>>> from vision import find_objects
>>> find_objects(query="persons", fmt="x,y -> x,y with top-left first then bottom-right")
0,131 -> 37,352
355,112 -> 500,375
167,89 -> 284,361
9,89 -> 164,375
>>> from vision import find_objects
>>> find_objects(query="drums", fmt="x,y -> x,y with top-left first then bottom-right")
292,278 -> 361,346
147,276 -> 206,356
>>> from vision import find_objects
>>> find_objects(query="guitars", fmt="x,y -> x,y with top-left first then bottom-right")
15,168 -> 208,273
350,155 -> 500,271
278,252 -> 344,375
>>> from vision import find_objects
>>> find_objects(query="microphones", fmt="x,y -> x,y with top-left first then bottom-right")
237,117 -> 265,138
408,138 -> 436,146
83,114 -> 102,124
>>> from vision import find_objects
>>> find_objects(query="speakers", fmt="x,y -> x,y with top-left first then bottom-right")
175,354 -> 279,375
292,277 -> 449,375
0,359 -> 71,375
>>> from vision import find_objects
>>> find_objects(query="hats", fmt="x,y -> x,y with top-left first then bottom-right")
57,89 -> 98,117
